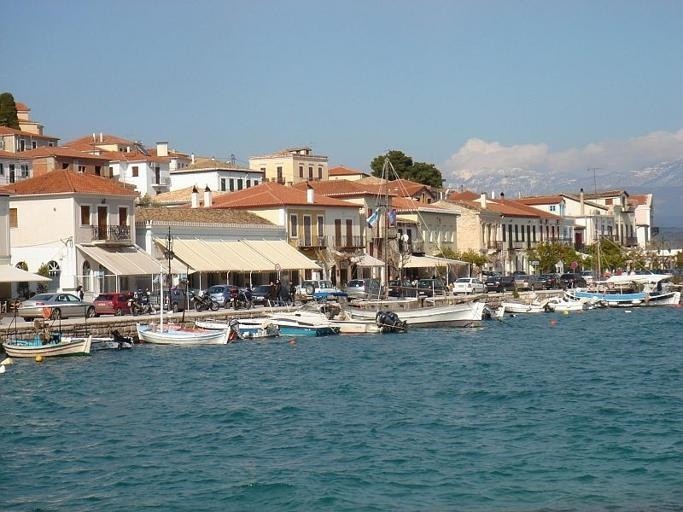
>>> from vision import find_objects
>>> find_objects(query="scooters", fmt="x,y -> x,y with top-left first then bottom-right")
127,288 -> 154,316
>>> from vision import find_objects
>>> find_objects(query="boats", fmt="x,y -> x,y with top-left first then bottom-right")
2,323 -> 94,358
485,292 -> 608,318
314,320 -> 384,333
61,335 -> 134,351
350,296 -> 483,328
195,317 -> 279,339
137,260 -> 231,347
264,310 -> 346,329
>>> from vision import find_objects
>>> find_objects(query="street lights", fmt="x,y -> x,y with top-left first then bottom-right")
165,225 -> 174,312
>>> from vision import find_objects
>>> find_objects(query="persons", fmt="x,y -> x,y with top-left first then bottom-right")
268,279 -> 296,306
75,286 -> 84,301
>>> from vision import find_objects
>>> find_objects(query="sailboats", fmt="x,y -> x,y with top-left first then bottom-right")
574,230 -> 683,307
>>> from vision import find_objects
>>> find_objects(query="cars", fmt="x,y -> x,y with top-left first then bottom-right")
147,279 -> 345,314
347,271 -> 594,297
92,293 -> 132,317
17,293 -> 96,322
